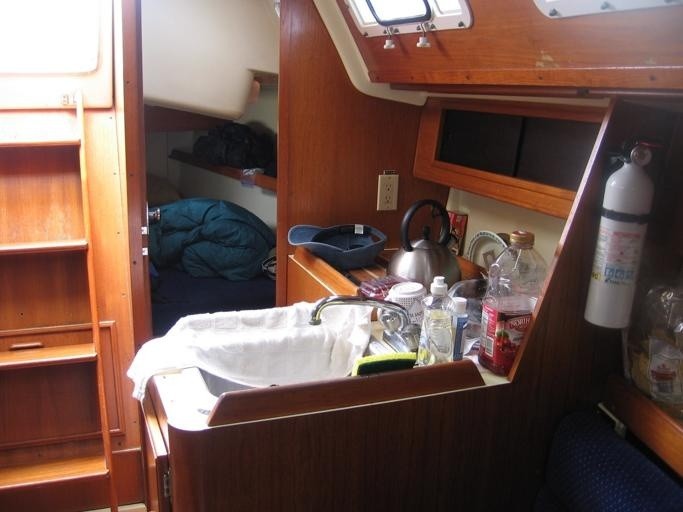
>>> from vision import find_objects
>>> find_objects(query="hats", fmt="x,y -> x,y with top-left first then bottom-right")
286,222 -> 386,269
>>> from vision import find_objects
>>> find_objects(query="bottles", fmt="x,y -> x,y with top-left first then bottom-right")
450,294 -> 469,362
416,273 -> 455,370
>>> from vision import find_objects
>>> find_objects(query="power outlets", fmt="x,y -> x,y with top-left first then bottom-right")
376,174 -> 399,212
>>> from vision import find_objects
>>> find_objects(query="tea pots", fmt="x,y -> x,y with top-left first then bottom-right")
385,198 -> 464,296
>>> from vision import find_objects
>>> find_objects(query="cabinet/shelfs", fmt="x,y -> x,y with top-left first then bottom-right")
411,95 -> 607,220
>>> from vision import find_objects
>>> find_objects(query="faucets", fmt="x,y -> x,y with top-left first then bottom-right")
310,295 -> 411,331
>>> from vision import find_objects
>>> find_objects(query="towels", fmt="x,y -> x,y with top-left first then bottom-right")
124,300 -> 373,401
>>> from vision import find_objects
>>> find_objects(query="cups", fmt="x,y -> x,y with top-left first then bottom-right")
376,281 -> 427,329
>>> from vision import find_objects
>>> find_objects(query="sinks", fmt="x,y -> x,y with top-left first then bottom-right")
190,337 -> 401,414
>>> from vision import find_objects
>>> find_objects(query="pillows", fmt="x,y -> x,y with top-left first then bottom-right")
147,172 -> 179,208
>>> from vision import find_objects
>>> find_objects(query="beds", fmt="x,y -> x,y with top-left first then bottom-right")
149,204 -> 276,336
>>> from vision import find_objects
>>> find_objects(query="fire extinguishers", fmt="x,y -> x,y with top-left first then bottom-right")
585,139 -> 664,329
